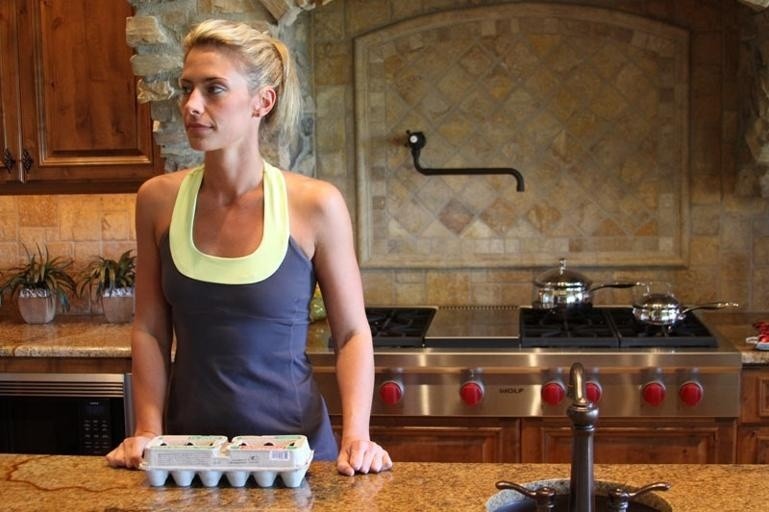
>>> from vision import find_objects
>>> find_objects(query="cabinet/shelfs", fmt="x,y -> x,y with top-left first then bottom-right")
0,0 -> 165,195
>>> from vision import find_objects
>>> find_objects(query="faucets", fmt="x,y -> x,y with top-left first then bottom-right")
563,362 -> 600,511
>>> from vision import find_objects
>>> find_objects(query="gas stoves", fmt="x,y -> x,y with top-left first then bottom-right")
327,305 -> 742,421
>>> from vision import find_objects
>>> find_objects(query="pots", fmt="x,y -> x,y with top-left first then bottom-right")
632,293 -> 739,329
532,256 -> 636,318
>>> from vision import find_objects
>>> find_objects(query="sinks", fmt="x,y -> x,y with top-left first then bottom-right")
485,478 -> 673,511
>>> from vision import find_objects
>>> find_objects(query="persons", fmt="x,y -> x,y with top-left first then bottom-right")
105,19 -> 393,476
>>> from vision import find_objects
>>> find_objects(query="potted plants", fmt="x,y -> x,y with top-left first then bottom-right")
0,242 -> 136,325
